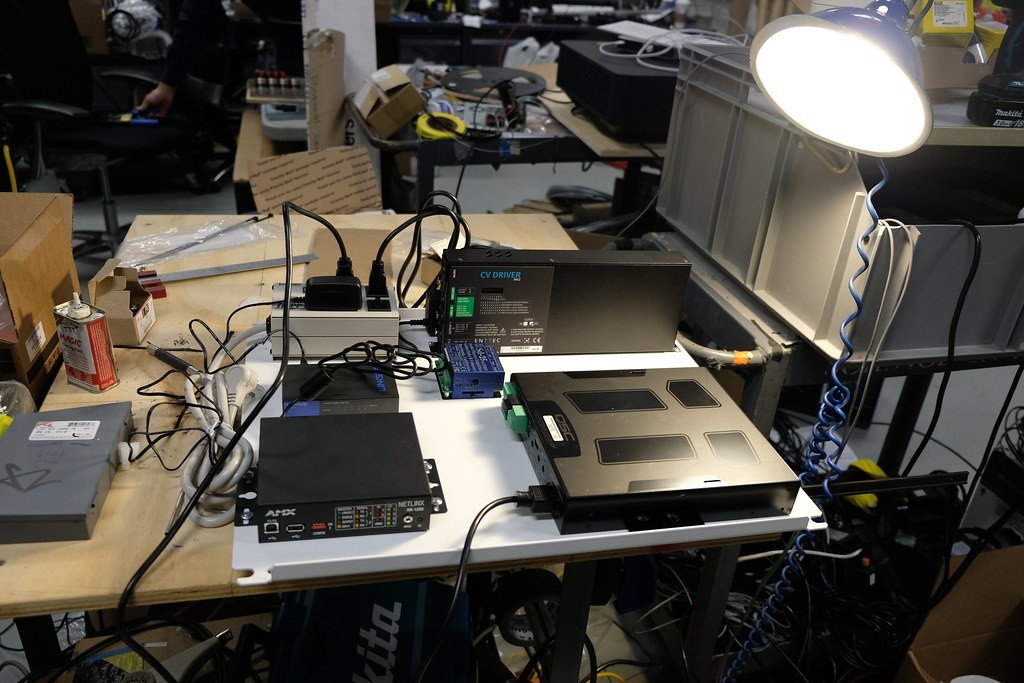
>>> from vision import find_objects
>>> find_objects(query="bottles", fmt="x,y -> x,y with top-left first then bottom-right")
0,380 -> 39,437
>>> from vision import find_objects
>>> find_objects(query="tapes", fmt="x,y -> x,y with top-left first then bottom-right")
417,112 -> 467,139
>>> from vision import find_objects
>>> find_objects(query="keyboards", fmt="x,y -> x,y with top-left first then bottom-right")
596,19 -> 730,50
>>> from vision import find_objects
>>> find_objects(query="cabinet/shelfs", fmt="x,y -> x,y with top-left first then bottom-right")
376,21 -> 621,67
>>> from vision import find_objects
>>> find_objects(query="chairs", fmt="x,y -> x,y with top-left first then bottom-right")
246,143 -> 384,215
0,0 -> 181,263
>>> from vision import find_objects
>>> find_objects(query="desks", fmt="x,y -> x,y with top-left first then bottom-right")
347,89 -> 667,215
0,213 -> 830,683
645,225 -> 1024,683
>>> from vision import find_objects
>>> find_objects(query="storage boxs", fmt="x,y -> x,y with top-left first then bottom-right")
54,612 -> 272,683
913,0 -> 1017,62
657,38 -> 1024,370
353,63 -> 428,139
0,192 -> 84,411
504,198 -> 650,251
89,258 -> 156,346
232,107 -> 280,213
891,545 -> 1024,683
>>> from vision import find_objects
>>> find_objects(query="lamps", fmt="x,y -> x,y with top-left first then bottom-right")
746,0 -> 935,157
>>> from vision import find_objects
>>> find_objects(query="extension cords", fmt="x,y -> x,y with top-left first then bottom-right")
270,283 -> 399,359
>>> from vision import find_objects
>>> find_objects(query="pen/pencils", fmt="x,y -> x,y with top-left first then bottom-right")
145,340 -> 200,374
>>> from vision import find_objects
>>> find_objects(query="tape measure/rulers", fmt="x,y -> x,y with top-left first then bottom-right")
136,253 -> 320,285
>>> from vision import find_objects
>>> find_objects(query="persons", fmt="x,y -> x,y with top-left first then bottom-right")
135,0 -> 258,180
